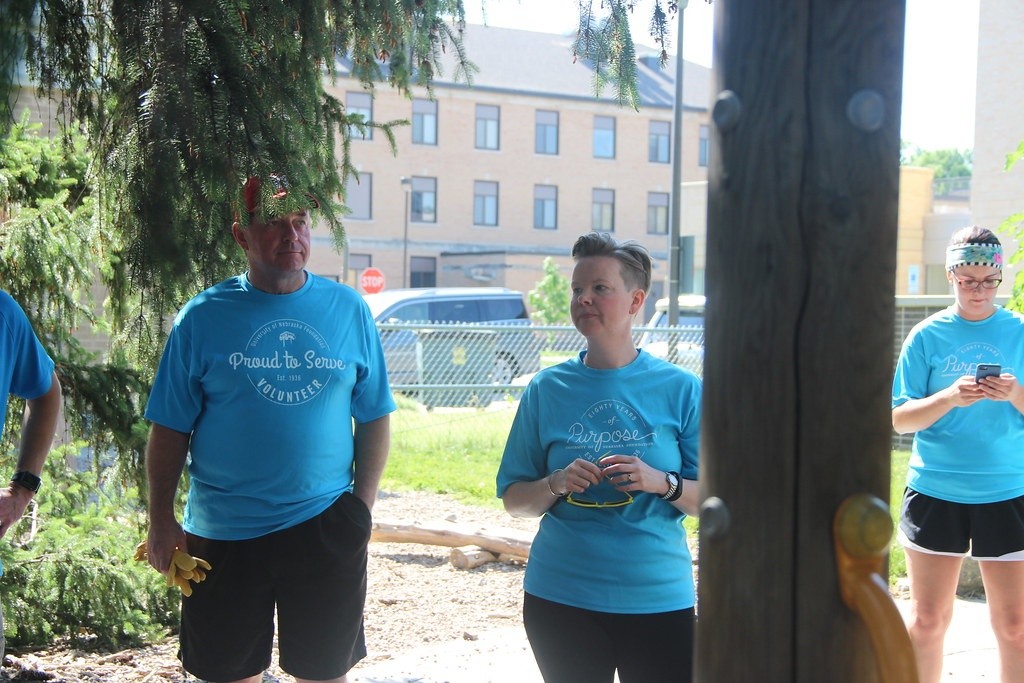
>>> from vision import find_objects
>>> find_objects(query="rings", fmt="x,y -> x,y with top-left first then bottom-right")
627,474 -> 631,484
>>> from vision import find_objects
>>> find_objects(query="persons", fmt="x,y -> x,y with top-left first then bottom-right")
0,289 -> 63,675
890,226 -> 1024,683
493,231 -> 703,683
142,174 -> 398,683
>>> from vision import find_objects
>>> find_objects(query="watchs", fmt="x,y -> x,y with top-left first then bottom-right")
11,471 -> 42,494
658,470 -> 678,500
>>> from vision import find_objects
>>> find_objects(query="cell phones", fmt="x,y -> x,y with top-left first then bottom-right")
975,364 -> 1002,384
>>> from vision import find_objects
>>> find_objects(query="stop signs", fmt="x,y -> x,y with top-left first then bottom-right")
360,268 -> 384,294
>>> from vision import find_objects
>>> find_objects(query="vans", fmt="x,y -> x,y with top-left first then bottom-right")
356,288 -> 541,393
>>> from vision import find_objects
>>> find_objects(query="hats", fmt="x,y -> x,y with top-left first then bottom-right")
233,175 -> 320,216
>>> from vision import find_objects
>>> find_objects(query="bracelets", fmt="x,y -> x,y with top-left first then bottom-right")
663,470 -> 683,503
548,470 -> 566,496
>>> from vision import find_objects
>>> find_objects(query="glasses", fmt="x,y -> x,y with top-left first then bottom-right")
950,267 -> 1003,289
564,450 -> 634,508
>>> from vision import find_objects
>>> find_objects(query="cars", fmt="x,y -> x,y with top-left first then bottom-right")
637,293 -> 704,381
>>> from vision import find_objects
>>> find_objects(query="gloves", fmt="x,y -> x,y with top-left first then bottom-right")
135,541 -> 212,595
